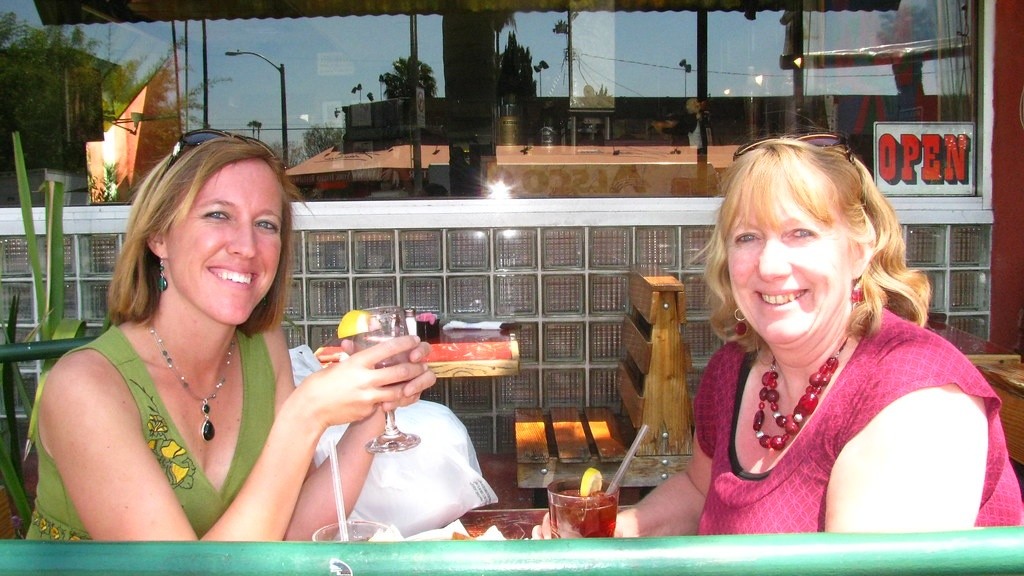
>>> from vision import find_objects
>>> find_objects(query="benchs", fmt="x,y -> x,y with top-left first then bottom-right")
978,364 -> 1024,469
511,269 -> 695,487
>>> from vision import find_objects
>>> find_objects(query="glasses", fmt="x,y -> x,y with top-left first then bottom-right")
161,129 -> 272,178
732,129 -> 851,169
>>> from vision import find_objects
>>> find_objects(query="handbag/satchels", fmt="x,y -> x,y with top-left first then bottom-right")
288,345 -> 500,541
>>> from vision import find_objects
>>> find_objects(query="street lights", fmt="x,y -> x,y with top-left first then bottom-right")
225,48 -> 289,168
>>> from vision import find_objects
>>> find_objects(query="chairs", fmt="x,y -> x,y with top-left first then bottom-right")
0,335 -> 98,532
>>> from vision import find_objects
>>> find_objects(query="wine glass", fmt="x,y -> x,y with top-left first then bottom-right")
352,306 -> 422,454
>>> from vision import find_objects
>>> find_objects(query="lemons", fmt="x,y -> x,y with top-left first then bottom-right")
337,310 -> 379,338
580,468 -> 602,496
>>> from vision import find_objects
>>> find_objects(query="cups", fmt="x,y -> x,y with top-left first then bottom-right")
311,521 -> 388,542
547,477 -> 619,539
416,319 -> 440,342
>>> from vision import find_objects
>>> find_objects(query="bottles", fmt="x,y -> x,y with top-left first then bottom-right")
403,309 -> 417,336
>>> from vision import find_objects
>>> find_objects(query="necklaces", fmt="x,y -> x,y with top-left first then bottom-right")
149,322 -> 234,442
752,333 -> 851,451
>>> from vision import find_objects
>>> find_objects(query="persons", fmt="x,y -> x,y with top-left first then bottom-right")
526,136 -> 1024,537
22,138 -> 438,544
449,146 -> 480,196
651,99 -> 702,146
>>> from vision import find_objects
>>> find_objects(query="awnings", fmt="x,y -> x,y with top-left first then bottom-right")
285,145 -> 450,186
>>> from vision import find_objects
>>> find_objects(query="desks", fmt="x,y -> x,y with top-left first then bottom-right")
930,323 -> 1021,363
313,334 -> 521,454
463,505 -> 634,540
